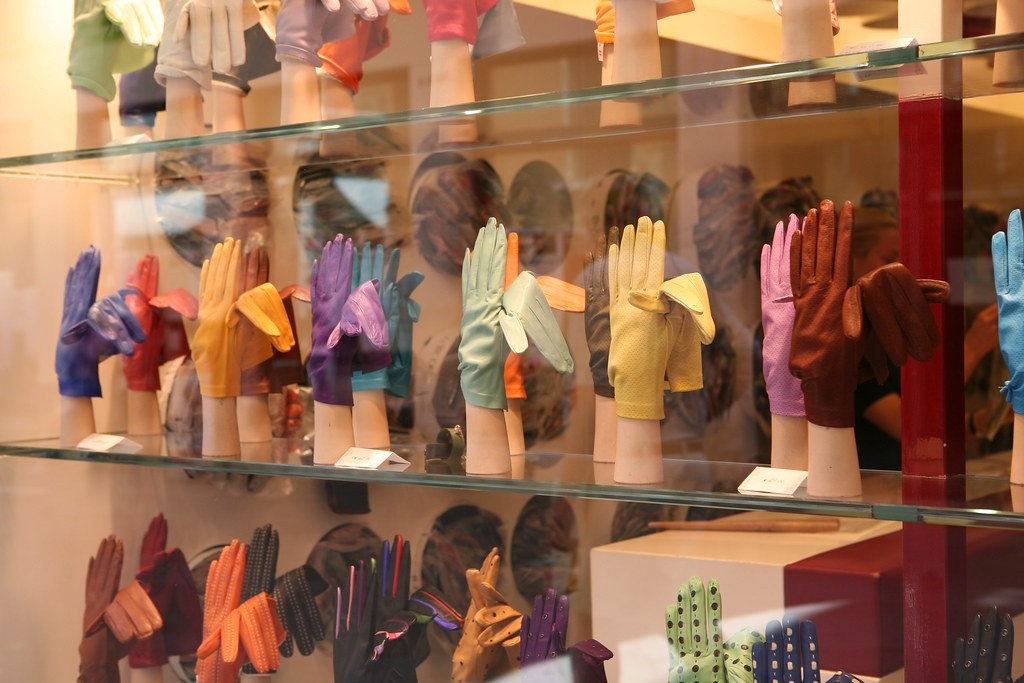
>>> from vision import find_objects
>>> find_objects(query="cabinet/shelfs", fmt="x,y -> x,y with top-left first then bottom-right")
0,25 -> 1024,683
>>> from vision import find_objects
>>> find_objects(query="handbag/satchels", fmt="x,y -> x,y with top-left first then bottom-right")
332,534 -> 464,683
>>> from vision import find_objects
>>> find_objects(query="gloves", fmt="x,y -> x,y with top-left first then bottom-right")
665,575 -> 765,683
607,216 -> 715,420
760,213 -> 807,418
69,0 -> 696,124
460,216 -> 620,409
77,512 -> 329,683
122,232 -> 426,407
787,199 -> 951,430
991,209 -> 1024,415
952,603 -> 1024,683
449,547 -> 523,683
519,589 -> 613,683
752,613 -> 852,683
56,245 -> 144,399
